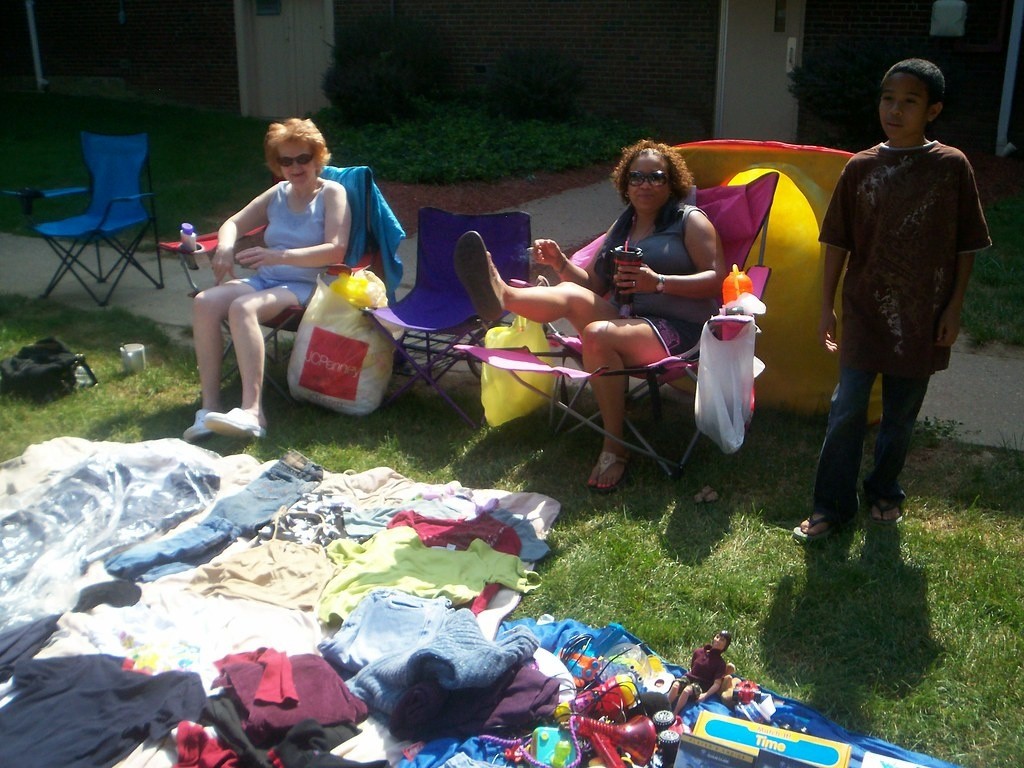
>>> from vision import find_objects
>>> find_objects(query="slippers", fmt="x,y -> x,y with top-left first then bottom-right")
869,500 -> 903,524
792,514 -> 853,542
205,407 -> 266,441
183,409 -> 214,441
453,231 -> 504,322
588,452 -> 629,493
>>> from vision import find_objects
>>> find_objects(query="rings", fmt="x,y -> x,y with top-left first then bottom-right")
632,281 -> 636,287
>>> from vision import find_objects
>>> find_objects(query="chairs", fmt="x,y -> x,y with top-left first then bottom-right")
0,133 -> 165,306
453,172 -> 779,481
158,168 -> 381,403
359,206 -> 531,430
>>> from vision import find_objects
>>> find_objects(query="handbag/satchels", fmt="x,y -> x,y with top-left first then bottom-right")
287,273 -> 395,415
0,337 -> 99,398
695,315 -> 754,454
480,315 -> 554,427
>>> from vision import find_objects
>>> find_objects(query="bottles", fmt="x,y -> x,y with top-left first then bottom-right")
75,353 -> 86,385
179,222 -> 200,271
721,271 -> 754,304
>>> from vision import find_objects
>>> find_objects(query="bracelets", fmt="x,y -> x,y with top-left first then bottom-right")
655,273 -> 666,295
555,255 -> 568,274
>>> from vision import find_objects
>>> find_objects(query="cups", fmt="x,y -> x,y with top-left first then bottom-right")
119,343 -> 146,374
233,235 -> 259,268
612,245 -> 643,304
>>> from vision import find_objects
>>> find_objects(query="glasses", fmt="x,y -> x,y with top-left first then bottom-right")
625,170 -> 672,188
276,154 -> 313,167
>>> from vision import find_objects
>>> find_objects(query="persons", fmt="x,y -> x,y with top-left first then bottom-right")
668,630 -> 732,718
183,118 -> 352,442
794,59 -> 993,549
453,140 -> 725,494
